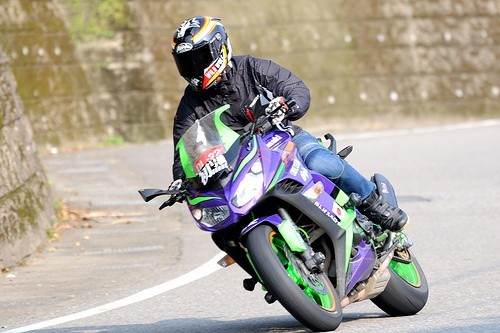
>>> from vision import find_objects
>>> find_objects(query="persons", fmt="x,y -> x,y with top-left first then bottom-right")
169,16 -> 408,303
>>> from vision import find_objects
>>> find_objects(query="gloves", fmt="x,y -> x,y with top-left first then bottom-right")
265,96 -> 288,118
166,178 -> 186,203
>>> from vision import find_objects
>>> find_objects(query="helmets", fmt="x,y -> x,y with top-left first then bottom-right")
173,16 -> 232,89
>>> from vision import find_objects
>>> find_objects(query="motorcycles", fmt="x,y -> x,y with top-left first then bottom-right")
138,97 -> 429,331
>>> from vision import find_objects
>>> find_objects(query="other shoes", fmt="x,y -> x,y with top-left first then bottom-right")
355,189 -> 409,231
